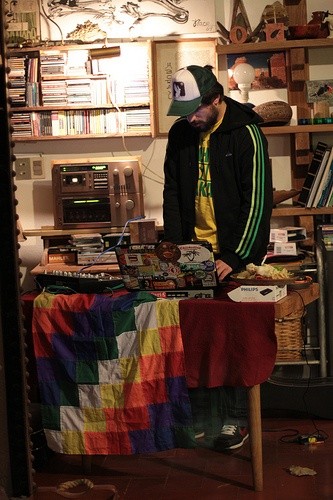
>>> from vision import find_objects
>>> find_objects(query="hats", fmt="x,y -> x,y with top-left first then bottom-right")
167,65 -> 217,117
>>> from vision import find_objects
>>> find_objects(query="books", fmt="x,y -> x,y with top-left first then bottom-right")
7,50 -> 151,136
298,141 -> 333,208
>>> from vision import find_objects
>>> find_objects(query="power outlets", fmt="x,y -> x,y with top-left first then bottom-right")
29,156 -> 50,179
14,157 -> 32,181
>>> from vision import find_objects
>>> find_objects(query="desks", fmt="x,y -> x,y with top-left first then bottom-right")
20,282 -> 319,492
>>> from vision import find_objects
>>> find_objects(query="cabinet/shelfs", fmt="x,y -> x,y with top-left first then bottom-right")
6,32 -> 333,379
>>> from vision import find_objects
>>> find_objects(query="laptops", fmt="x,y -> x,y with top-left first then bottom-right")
114,240 -> 226,291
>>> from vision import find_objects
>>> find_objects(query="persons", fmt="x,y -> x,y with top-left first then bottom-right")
162,65 -> 272,449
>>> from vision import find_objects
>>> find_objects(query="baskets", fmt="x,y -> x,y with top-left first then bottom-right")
275,319 -> 304,362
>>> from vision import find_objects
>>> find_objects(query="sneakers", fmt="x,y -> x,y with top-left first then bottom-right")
215,424 -> 249,450
195,432 -> 205,439
